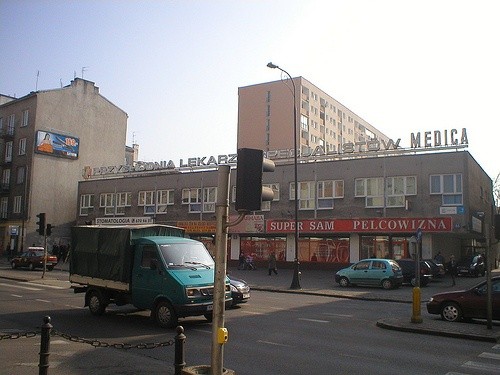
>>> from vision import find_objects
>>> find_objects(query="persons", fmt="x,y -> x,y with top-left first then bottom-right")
52,241 -> 68,262
435,251 -> 445,262
448,255 -> 458,286
246,252 -> 256,271
6,242 -> 12,262
239,252 -> 245,273
472,253 -> 484,265
268,251 -> 278,275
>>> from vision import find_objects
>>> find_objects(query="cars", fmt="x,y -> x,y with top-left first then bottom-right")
425,274 -> 500,323
227,271 -> 252,306
454,253 -> 486,278
9,246 -> 58,271
393,257 -> 437,287
432,260 -> 449,276
333,257 -> 404,290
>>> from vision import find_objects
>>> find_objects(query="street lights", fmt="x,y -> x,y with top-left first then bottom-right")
266,61 -> 301,290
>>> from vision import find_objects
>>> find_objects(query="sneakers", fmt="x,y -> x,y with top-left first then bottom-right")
266,273 -> 280,276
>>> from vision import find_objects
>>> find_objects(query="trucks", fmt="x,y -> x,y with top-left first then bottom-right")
68,224 -> 234,330
